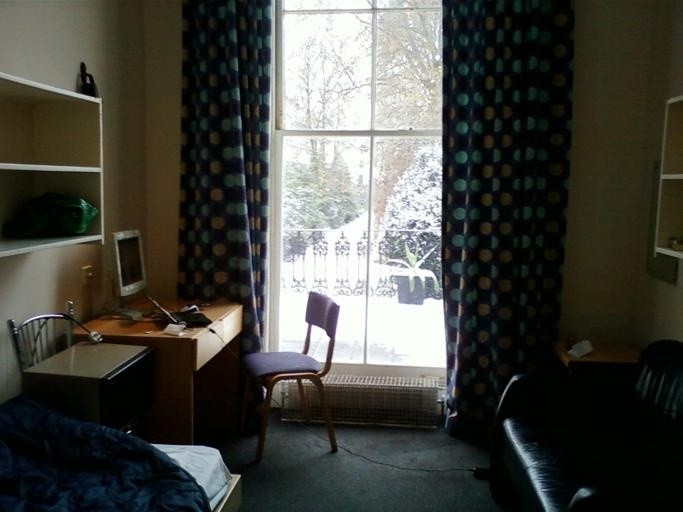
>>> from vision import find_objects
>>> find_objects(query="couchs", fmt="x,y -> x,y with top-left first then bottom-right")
495,341 -> 682,511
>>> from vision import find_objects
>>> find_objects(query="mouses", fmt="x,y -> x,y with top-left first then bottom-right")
180,304 -> 199,312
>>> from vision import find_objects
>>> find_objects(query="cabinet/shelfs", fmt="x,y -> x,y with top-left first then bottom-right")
653,93 -> 683,265
552,332 -> 639,375
0,70 -> 107,257
23,340 -> 153,445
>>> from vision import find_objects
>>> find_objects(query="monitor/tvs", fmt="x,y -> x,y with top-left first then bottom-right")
104,229 -> 148,320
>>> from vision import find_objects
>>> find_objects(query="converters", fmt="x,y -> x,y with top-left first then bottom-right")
474,468 -> 487,479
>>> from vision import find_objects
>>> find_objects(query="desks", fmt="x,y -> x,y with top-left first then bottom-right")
72,293 -> 245,444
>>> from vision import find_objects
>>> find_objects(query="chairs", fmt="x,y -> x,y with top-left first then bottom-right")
247,291 -> 340,466
8,299 -> 83,413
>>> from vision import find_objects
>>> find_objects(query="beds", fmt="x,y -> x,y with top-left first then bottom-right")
0,445 -> 242,512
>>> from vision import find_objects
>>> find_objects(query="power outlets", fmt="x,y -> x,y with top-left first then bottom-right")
104,267 -> 114,287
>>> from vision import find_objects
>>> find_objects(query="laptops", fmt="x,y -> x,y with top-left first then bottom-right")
143,292 -> 213,327
